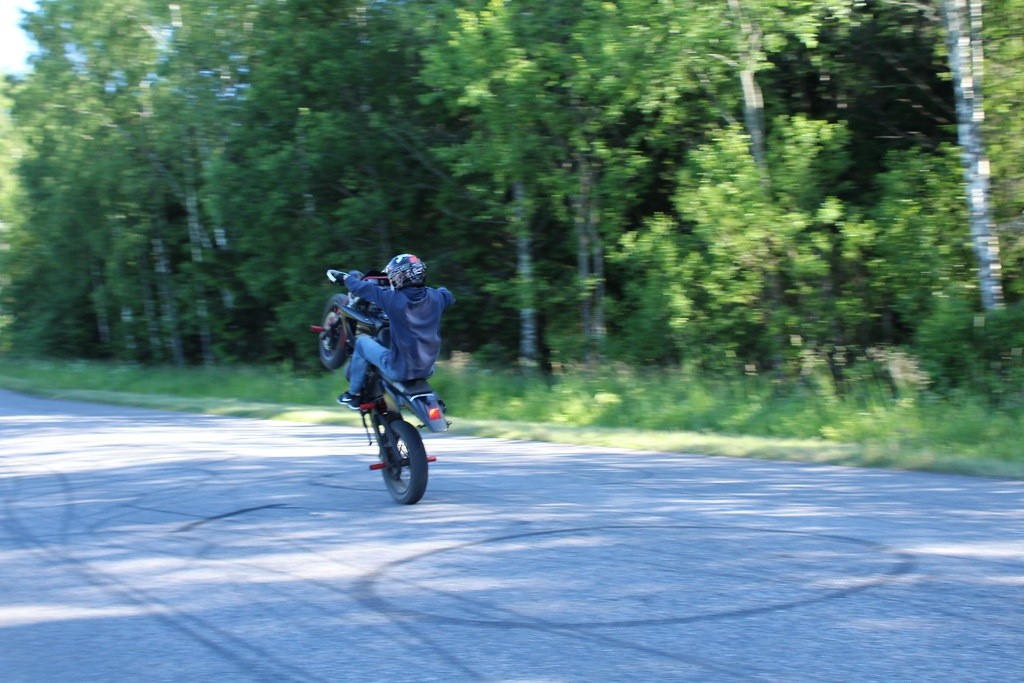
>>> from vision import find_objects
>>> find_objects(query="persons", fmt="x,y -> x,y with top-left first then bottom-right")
328,253 -> 455,410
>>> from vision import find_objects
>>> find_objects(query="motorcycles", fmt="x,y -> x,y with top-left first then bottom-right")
310,268 -> 454,504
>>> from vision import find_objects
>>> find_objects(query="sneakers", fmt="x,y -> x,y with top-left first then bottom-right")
336,392 -> 361,410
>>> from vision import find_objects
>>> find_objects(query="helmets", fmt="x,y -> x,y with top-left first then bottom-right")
381,253 -> 427,290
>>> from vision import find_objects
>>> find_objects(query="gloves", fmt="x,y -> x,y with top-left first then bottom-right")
331,271 -> 345,286
